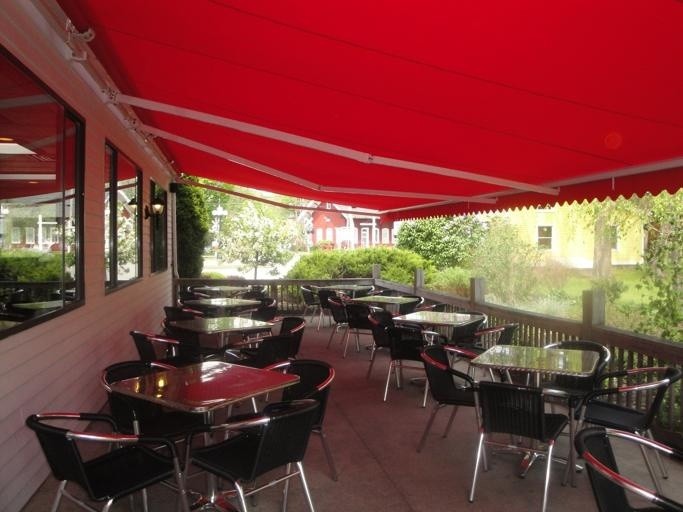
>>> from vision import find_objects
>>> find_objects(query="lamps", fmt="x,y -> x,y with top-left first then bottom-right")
144,194 -> 165,230
128,198 -> 138,217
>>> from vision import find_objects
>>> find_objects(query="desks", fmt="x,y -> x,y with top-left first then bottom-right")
0,320 -> 21,329
12,300 -> 61,310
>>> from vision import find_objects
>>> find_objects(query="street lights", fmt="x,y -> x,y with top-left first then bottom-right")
211,206 -> 228,260
